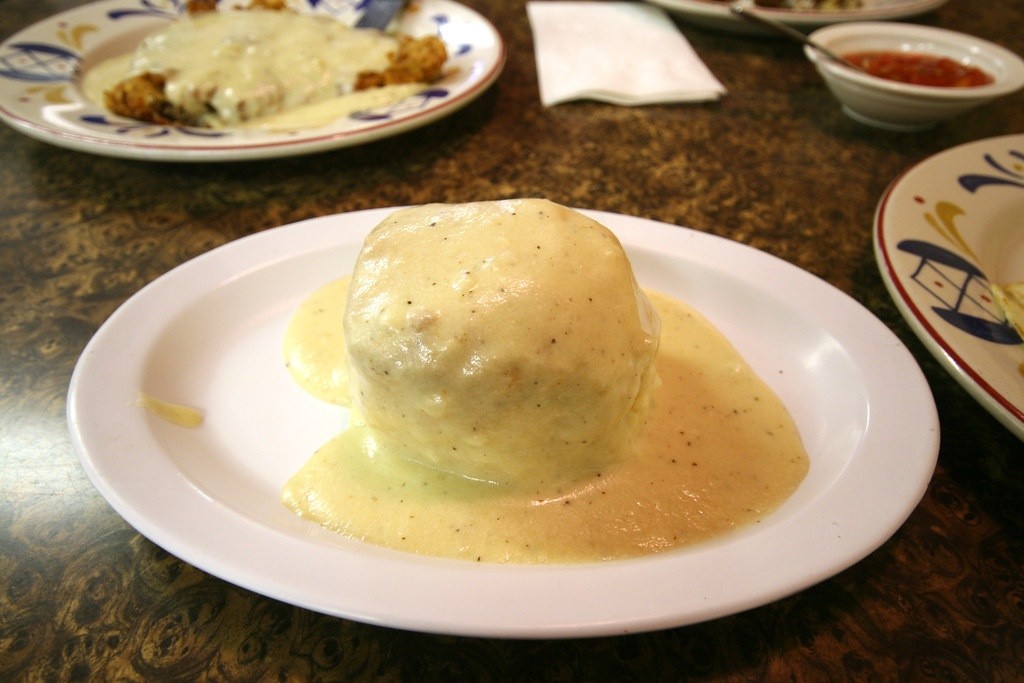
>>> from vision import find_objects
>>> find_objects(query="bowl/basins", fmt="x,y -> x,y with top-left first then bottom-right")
797,23 -> 1024,133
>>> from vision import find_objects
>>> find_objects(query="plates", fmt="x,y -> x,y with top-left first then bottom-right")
872,133 -> 1023,444
0,0 -> 505,162
65,206 -> 941,638
646,1 -> 945,33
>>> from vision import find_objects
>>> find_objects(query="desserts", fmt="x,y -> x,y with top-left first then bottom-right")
277,197 -> 813,567
